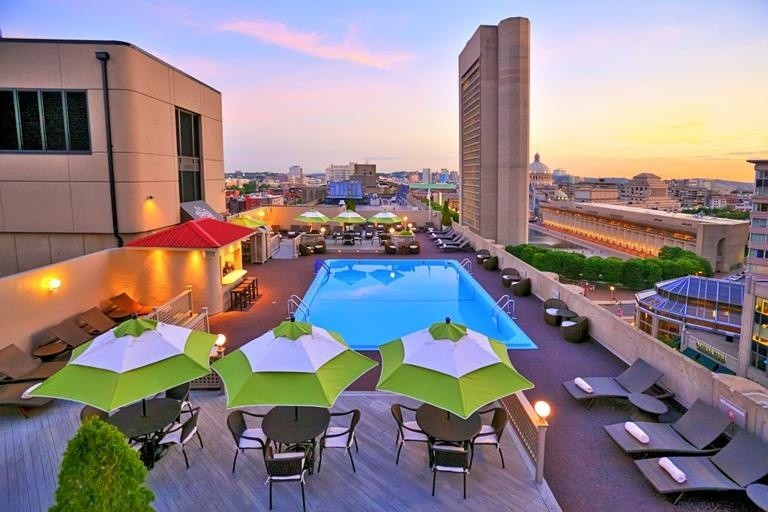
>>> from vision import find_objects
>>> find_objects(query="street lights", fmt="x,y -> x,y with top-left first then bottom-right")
610,287 -> 614,300
320,227 -> 325,234
407,223 -> 413,230
215,334 -> 225,394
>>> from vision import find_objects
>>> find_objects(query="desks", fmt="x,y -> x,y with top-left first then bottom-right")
508,275 -> 520,286
415,402 -> 482,468
557,309 -> 578,321
628,393 -> 668,422
746,483 -> 768,512
80,398 -> 181,462
32,342 -> 67,362
261,405 -> 331,474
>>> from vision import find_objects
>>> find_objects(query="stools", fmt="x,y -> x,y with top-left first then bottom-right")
239,277 -> 258,303
230,288 -> 246,311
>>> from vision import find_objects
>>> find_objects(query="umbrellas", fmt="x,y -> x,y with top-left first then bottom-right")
21,319 -> 217,412
292,209 -> 330,231
229,215 -> 268,233
368,211 -> 403,233
211,315 -> 378,420
375,317 -> 533,418
333,209 -> 367,233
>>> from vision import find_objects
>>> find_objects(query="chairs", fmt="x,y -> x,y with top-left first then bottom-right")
543,298 -> 568,326
501,268 -> 520,288
602,398 -> 733,458
317,409 -> 361,473
511,278 -> 531,297
0,344 -> 65,380
432,443 -> 470,499
79,307 -> 118,333
109,292 -> 155,315
153,382 -> 193,423
560,316 -> 588,343
227,410 -> 277,474
215,334 -> 226,350
467,408 -> 508,469
48,319 -> 93,348
634,428 -> 768,504
561,357 -> 664,409
266,445 -> 311,512
271,224 -> 470,255
475,249 -> 499,271
151,407 -> 203,469
0,382 -> 55,418
390,403 -> 434,467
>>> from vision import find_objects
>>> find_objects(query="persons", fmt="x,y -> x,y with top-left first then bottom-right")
223,262 -> 233,274
